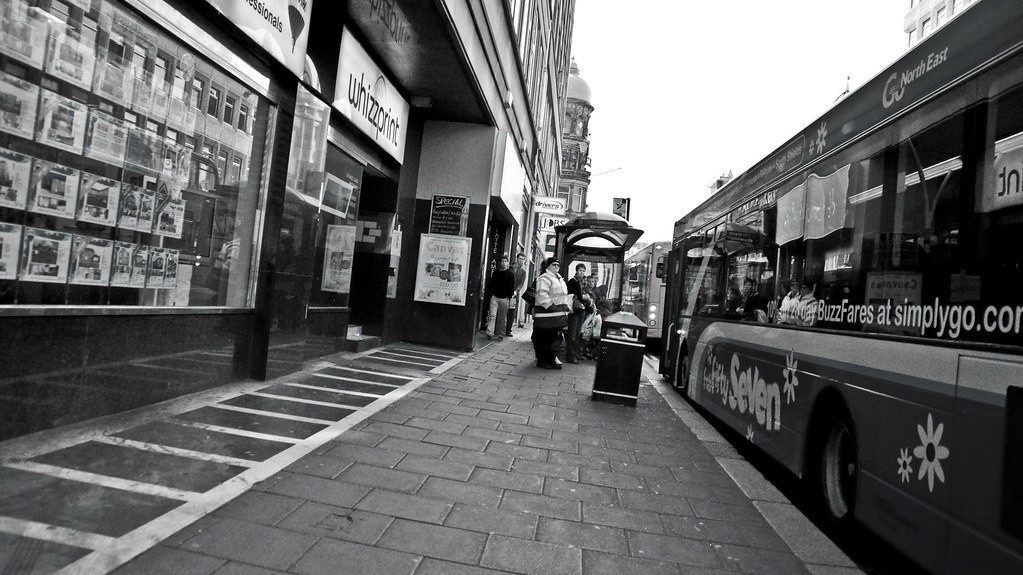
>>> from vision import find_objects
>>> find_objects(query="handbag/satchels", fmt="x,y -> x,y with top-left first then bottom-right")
522,278 -> 537,304
580,311 -> 602,341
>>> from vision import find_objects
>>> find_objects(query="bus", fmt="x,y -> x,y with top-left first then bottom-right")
622,241 -> 717,338
654,0 -> 1023,575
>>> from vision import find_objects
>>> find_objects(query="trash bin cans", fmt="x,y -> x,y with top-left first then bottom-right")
590,311 -> 648,408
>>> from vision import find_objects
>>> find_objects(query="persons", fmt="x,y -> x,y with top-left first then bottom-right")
205,225 -> 241,306
734,276 -> 818,326
485,252 -> 527,340
566,264 -> 609,364
528,257 -> 572,370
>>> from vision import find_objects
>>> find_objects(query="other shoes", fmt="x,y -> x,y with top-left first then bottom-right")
506,332 -> 513,337
536,361 -> 562,369
499,333 -> 504,339
576,354 -> 587,361
487,333 -> 492,340
566,357 -> 578,364
555,356 -> 563,365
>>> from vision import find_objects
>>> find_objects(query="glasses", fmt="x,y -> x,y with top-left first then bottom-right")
800,286 -> 808,290
551,263 -> 560,267
791,284 -> 800,287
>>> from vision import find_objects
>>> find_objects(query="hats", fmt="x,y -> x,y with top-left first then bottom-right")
547,257 -> 560,268
791,277 -> 800,284
